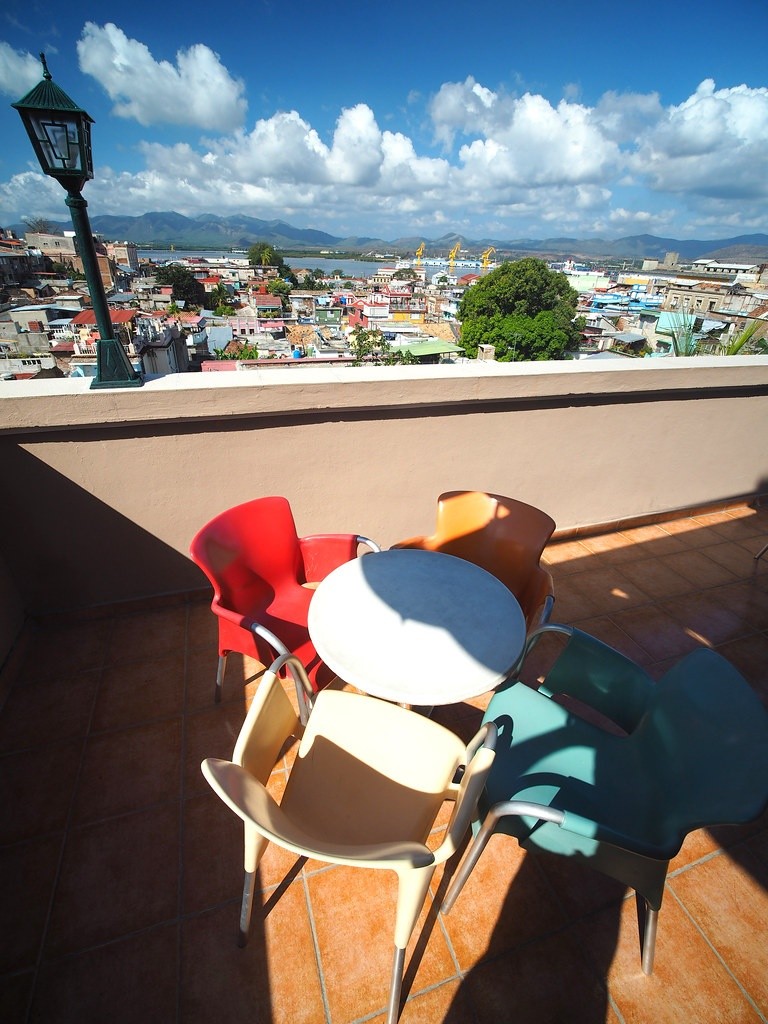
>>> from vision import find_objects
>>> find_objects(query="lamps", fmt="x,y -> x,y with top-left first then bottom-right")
6,50 -> 145,389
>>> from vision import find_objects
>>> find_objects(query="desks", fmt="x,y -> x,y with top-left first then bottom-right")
307,548 -> 526,719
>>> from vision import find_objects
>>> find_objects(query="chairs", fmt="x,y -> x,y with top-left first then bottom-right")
201,654 -> 497,1024
389,491 -> 556,682
438,622 -> 768,976
189,496 -> 381,726
